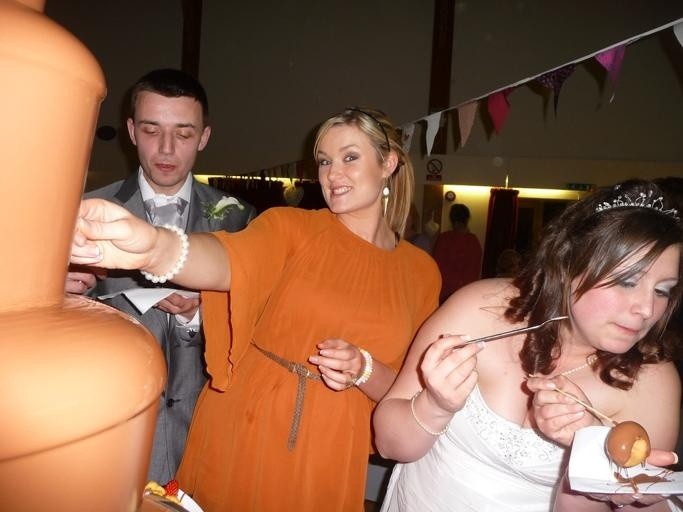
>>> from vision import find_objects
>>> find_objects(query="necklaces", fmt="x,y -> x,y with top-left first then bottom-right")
558,353 -> 599,376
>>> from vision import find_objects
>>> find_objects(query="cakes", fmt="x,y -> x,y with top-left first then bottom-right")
0,0 -> 173,512
604,421 -> 651,467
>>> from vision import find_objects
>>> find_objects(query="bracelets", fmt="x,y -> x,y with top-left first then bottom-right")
410,392 -> 451,435
353,345 -> 373,389
141,221 -> 190,284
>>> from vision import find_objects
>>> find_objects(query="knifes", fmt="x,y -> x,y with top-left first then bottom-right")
437,316 -> 571,353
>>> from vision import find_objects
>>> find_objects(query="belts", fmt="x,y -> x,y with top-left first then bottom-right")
250,338 -> 325,448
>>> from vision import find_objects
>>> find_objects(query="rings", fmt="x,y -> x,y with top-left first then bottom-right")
348,372 -> 356,387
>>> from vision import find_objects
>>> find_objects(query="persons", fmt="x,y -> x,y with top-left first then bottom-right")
372,180 -> 683,512
404,203 -> 430,249
70,105 -> 444,512
434,204 -> 482,304
527,375 -> 683,512
65,67 -> 258,488
